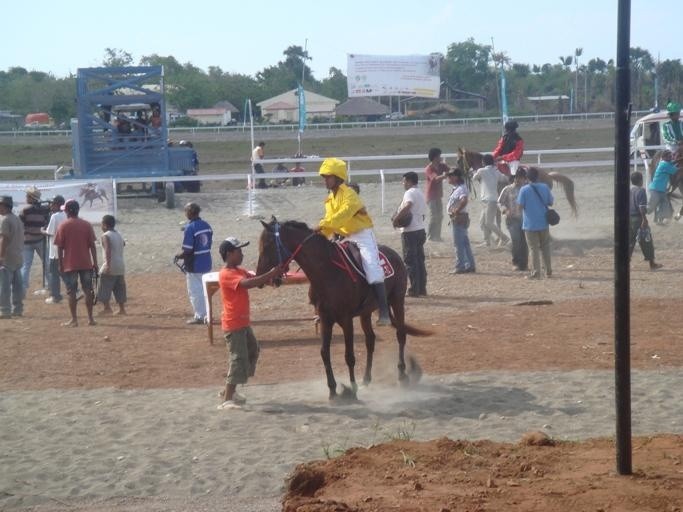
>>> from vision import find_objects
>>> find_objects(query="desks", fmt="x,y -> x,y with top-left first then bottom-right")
202,272 -> 320,346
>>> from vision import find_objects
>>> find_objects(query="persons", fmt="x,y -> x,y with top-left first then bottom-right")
446,168 -> 475,274
269,163 -> 289,187
290,162 -> 306,188
661,102 -> 683,152
314,157 -> 392,327
96,215 -> 128,314
0,195 -> 24,319
473,155 -> 510,248
40,196 -> 67,305
19,185 -> 49,289
390,172 -> 427,297
517,168 -> 554,279
492,119 -> 524,176
423,147 -> 449,241
175,202 -> 213,324
628,171 -> 664,271
53,199 -> 97,326
218,237 -> 280,409
646,149 -> 677,219
116,109 -> 160,143
251,142 -> 265,187
497,168 -> 529,271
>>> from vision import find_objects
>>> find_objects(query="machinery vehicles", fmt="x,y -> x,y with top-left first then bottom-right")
67,65 -> 193,208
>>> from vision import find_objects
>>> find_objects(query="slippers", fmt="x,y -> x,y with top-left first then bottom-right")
217,389 -> 247,403
650,264 -> 664,271
217,399 -> 242,410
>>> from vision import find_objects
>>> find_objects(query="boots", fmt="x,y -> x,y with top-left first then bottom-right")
372,281 -> 393,327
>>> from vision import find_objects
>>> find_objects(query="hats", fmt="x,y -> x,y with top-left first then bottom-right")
319,158 -> 347,182
59,198 -> 79,211
49,194 -> 65,205
23,184 -> 41,201
666,101 -> 681,114
218,237 -> 250,258
504,119 -> 519,130
446,167 -> 461,176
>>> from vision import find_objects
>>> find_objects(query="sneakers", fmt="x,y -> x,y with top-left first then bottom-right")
45,296 -> 59,303
498,236 -> 510,246
476,240 -> 491,247
187,317 -> 204,324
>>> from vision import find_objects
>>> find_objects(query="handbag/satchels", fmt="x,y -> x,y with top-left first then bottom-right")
530,183 -> 560,226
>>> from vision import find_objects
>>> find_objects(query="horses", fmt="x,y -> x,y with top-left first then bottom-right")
255,215 -> 432,402
78,187 -> 109,208
456,145 -> 579,243
649,148 -> 683,223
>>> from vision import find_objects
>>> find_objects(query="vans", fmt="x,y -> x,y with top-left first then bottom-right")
631,109 -> 683,160
